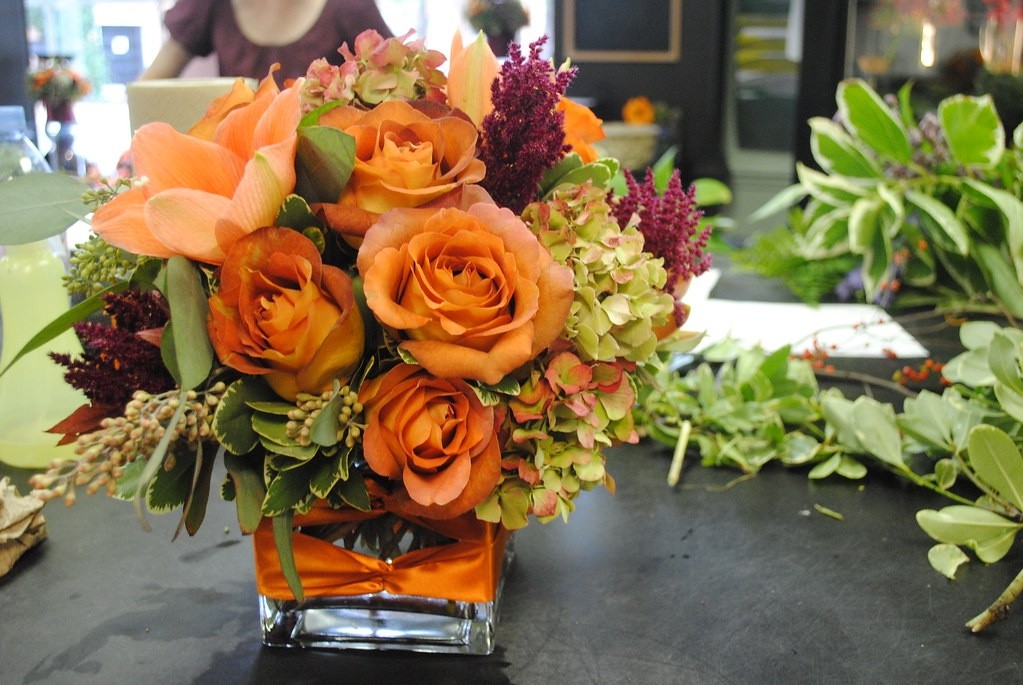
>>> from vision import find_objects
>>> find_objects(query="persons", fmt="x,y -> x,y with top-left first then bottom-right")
139,0 -> 395,93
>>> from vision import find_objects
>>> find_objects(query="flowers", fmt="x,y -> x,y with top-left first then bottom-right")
23,59 -> 90,109
0,4 -> 704,602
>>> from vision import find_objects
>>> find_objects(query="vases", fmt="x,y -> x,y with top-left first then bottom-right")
39,95 -> 77,139
251,460 -> 521,656
